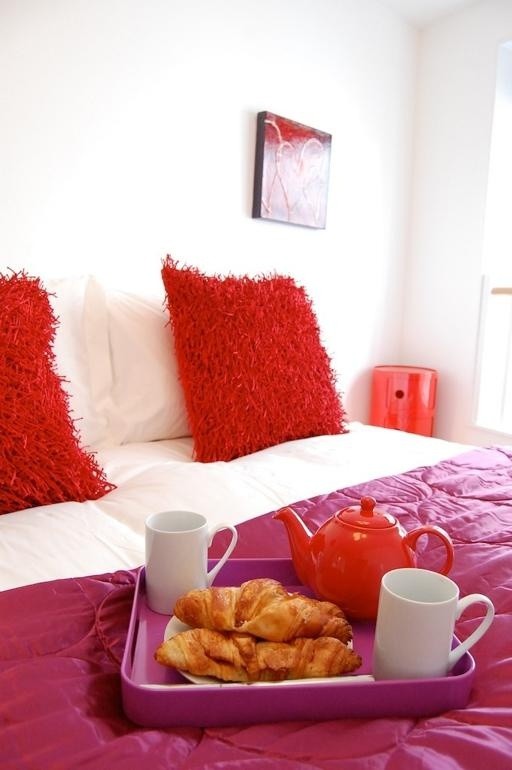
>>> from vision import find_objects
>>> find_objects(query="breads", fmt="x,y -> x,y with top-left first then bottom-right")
154,578 -> 363,683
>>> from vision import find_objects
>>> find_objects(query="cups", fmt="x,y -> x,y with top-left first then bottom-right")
374,567 -> 483,675
143,512 -> 238,615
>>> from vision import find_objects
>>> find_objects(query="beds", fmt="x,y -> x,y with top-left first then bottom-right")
0,410 -> 512,770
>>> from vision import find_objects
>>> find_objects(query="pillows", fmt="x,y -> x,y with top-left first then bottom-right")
1,272 -> 115,516
159,257 -> 349,464
70,271 -> 193,449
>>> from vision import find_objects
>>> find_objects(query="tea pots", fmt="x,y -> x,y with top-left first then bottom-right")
273,496 -> 454,625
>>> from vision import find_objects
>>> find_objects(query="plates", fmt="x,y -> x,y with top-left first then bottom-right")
165,586 -> 353,686
